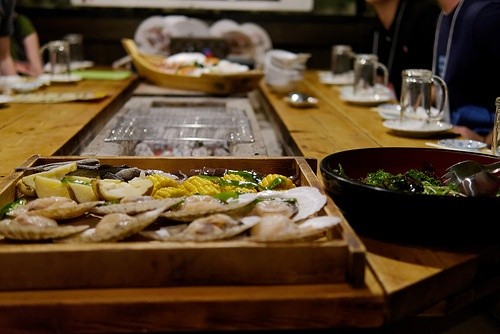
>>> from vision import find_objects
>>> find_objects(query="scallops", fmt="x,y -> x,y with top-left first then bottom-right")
0,186 -> 343,242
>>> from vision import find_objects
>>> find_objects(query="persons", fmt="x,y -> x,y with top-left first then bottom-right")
10,14 -> 43,76
0,0 -> 17,76
367,0 -> 440,104
430,0 -> 500,148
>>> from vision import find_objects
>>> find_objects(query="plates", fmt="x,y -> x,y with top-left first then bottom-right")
381,119 -> 453,137
319,72 -> 359,85
339,88 -> 391,105
120,35 -> 265,97
379,104 -> 444,120
437,138 -> 488,150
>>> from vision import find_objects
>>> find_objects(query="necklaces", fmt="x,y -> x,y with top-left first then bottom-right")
372,9 -> 402,73
432,0 -> 464,80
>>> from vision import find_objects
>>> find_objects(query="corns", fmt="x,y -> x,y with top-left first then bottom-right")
143,175 -> 295,200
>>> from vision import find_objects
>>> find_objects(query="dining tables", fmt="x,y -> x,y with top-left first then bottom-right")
0,62 -> 500,331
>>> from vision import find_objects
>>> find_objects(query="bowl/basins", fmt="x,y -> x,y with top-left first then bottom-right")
263,50 -> 313,94
320,142 -> 500,253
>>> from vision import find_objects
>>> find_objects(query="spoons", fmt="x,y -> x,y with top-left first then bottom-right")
440,157 -> 500,196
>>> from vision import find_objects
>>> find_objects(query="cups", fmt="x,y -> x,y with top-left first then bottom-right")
39,40 -> 73,78
352,53 -> 389,102
399,69 -> 448,130
66,33 -> 84,65
331,43 -> 358,83
